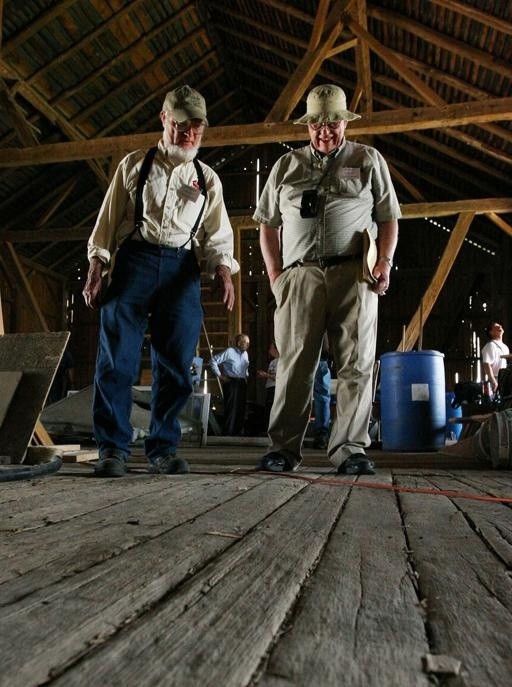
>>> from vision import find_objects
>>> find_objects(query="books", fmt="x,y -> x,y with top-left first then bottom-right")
358,229 -> 387,297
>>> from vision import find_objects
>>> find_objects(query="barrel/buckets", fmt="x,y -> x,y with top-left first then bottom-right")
445,392 -> 463,446
190,356 -> 204,386
479,407 -> 512,468
380,350 -> 446,452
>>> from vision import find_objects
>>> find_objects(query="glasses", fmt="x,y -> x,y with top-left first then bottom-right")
165,112 -> 207,136
309,122 -> 342,131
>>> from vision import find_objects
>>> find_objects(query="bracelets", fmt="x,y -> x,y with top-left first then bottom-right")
379,255 -> 393,267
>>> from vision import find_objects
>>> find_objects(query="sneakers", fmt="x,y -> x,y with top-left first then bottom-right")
338,452 -> 376,475
256,452 -> 292,472
94,452 -> 128,478
146,454 -> 191,475
313,430 -> 329,449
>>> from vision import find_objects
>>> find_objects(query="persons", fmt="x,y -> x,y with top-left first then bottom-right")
252,85 -> 402,476
481,322 -> 510,402
252,342 -> 280,436
212,332 -> 251,436
86,86 -> 243,474
314,357 -> 332,450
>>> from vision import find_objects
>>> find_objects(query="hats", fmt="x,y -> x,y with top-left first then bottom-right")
161,85 -> 210,126
293,84 -> 362,126
